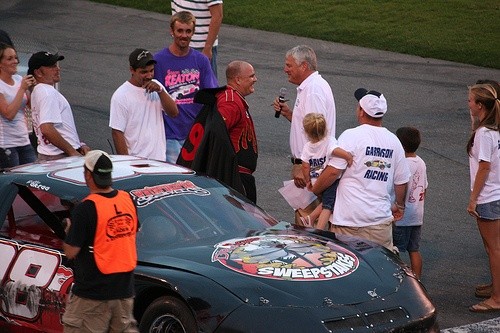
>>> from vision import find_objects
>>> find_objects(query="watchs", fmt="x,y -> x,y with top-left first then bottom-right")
156,86 -> 163,93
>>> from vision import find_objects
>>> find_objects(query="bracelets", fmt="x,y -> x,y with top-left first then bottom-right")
395,201 -> 406,210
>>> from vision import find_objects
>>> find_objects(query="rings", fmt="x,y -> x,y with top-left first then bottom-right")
469,213 -> 472,216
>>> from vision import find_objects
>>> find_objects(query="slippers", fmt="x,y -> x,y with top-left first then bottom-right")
474,285 -> 493,297
469,302 -> 500,312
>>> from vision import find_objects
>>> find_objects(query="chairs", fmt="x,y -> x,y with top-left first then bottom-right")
140,216 -> 181,247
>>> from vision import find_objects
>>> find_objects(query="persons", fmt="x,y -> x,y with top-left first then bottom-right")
60,150 -> 142,333
152,11 -> 220,164
0,28 -> 38,170
300,112 -> 353,231
468,79 -> 500,311
27,51 -> 91,159
175,60 -> 259,205
313,88 -> 412,255
274,44 -> 336,227
108,48 -> 179,162
170,0 -> 224,78
393,126 -> 428,278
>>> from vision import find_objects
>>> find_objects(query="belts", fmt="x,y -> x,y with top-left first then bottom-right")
290,157 -> 302,164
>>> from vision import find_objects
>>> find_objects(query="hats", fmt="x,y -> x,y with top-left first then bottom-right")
354,88 -> 388,118
129,48 -> 158,68
83,149 -> 113,173
28,51 -> 65,69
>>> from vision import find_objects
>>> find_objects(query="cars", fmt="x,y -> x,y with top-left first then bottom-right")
0,152 -> 438,333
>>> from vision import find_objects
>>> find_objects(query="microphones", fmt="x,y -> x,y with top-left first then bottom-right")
274,87 -> 287,119
